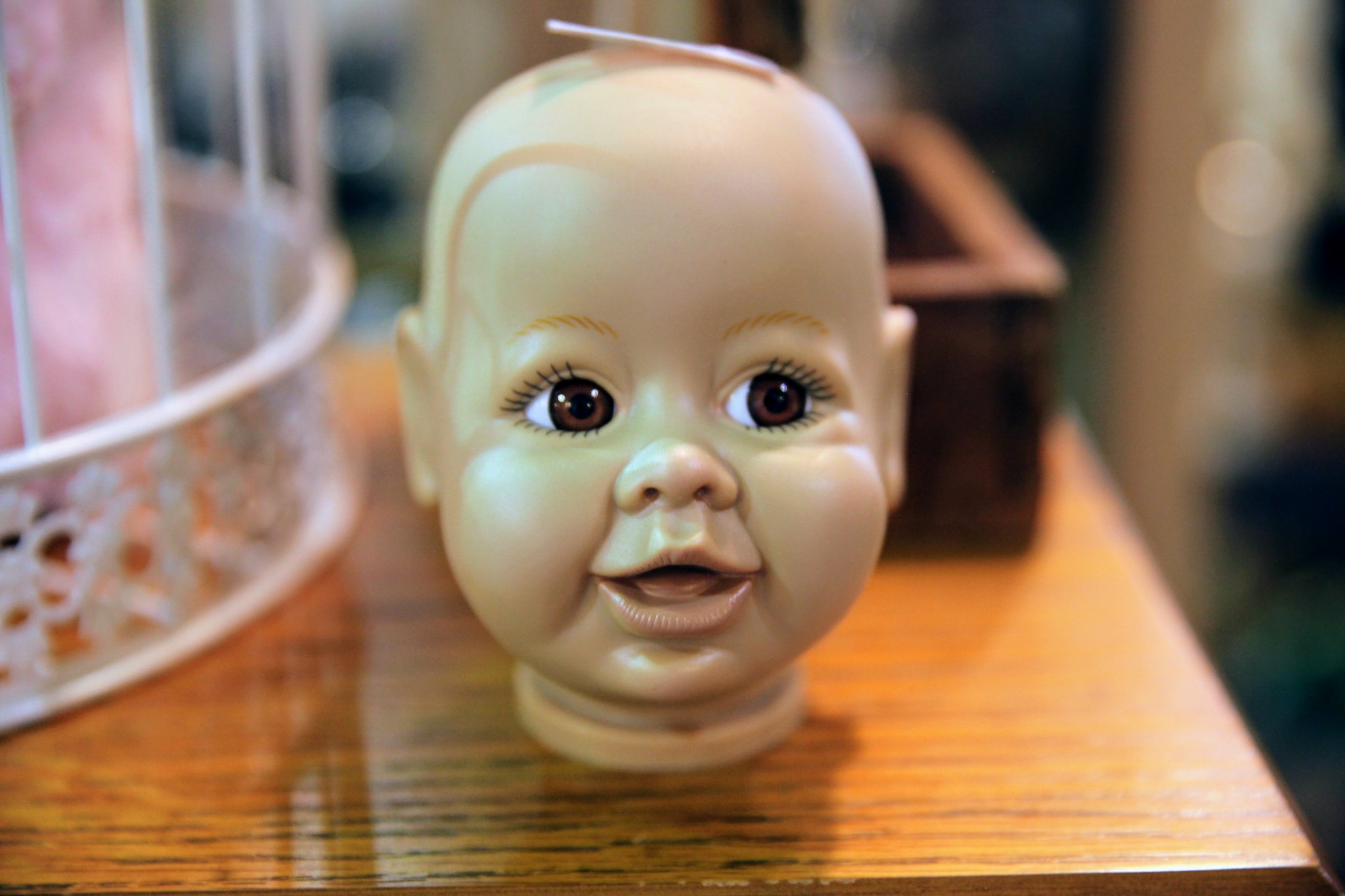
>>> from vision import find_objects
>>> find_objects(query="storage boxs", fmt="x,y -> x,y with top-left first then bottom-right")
859,107 -> 1065,571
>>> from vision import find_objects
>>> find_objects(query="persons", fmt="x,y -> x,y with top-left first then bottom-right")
388,42 -> 917,777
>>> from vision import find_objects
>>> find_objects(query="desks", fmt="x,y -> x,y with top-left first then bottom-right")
0,342 -> 1324,896
